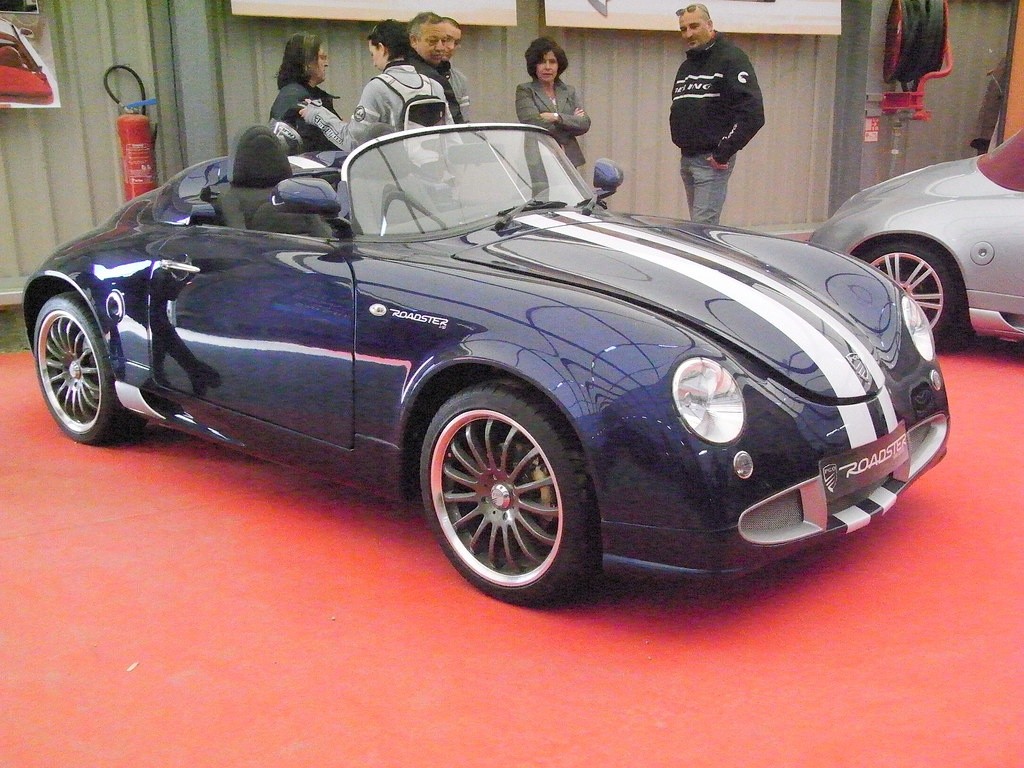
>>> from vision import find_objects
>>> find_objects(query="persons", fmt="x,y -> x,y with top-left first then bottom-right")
299,18 -> 465,181
407,11 -> 473,143
670,4 -> 765,225
515,36 -> 592,201
269,32 -> 345,155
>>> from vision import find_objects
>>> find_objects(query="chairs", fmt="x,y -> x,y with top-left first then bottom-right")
218,124 -> 323,236
348,123 -> 443,235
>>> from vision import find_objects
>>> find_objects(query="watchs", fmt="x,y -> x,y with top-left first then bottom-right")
554,112 -> 559,122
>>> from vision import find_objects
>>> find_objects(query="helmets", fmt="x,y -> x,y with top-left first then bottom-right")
266,118 -> 304,158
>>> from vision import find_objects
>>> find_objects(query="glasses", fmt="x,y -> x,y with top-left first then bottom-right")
676,4 -> 710,19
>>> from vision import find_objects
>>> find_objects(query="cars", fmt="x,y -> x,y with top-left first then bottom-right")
804,121 -> 1024,357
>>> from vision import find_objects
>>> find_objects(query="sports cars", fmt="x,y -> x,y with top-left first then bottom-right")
20,128 -> 951,614
0,13 -> 59,107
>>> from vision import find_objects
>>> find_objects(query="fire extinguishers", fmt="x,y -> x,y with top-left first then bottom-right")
115,97 -> 159,204
